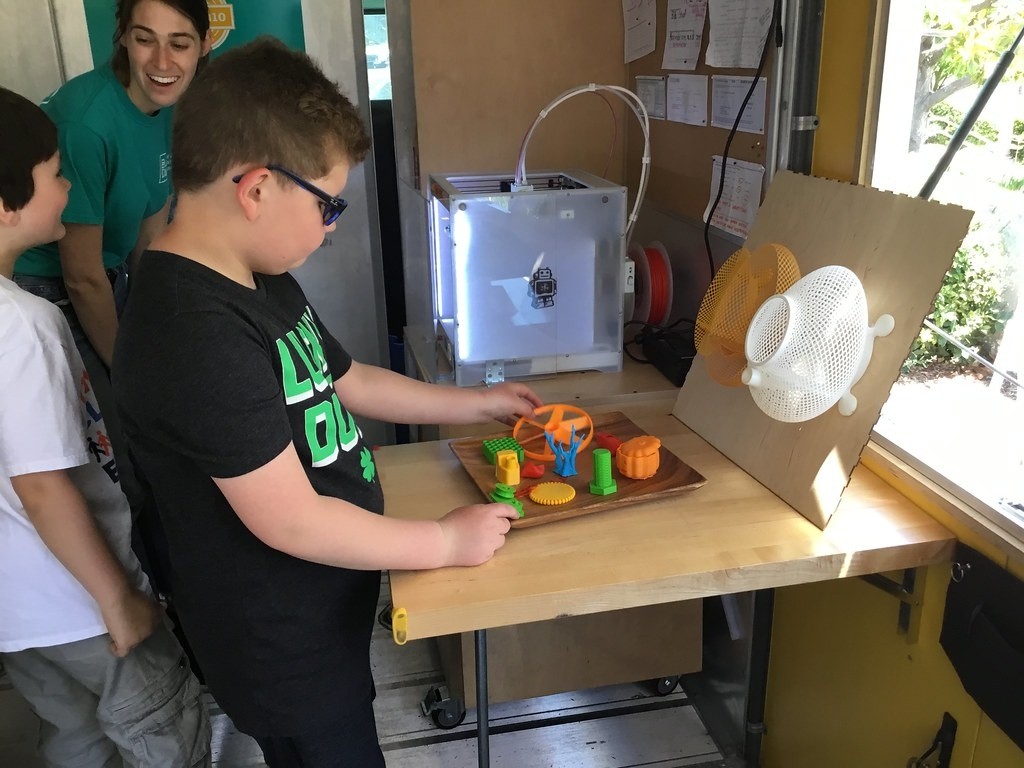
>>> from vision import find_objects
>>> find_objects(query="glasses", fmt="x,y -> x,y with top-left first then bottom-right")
232,163 -> 350,226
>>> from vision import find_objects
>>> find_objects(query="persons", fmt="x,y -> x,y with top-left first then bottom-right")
0,0 -> 215,589
112,34 -> 545,768
0,84 -> 212,768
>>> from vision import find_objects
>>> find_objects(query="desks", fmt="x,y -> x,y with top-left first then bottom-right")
371,380 -> 956,767
